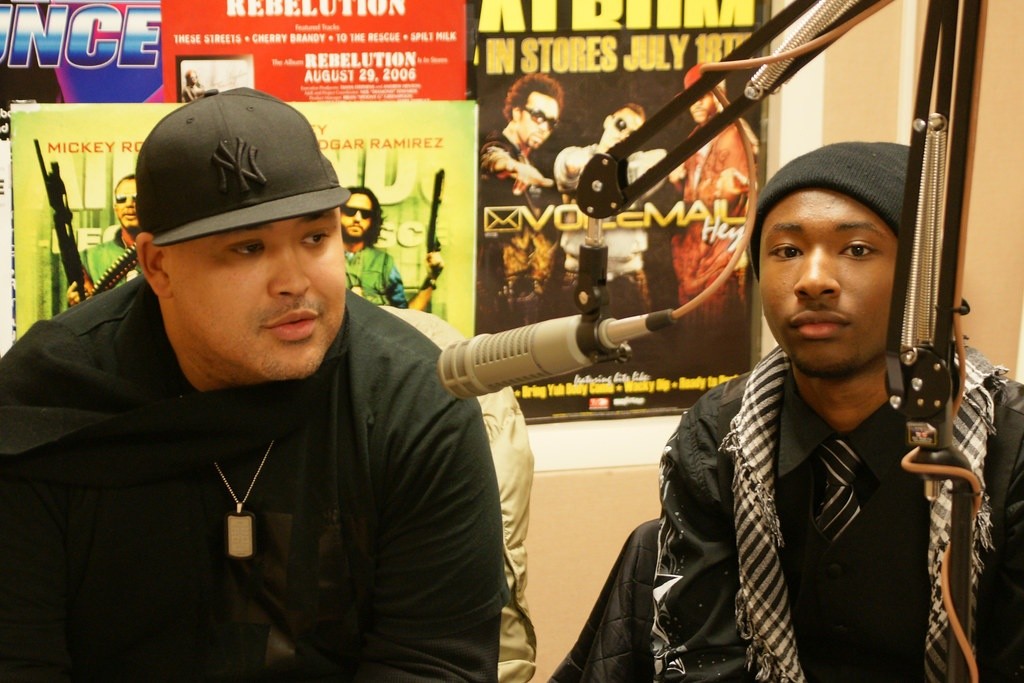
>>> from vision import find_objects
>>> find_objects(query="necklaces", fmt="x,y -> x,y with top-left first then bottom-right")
215,440 -> 275,558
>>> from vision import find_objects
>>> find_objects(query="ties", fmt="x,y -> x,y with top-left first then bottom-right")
815,439 -> 861,541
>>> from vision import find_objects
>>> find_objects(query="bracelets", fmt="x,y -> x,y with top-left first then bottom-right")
425,277 -> 437,290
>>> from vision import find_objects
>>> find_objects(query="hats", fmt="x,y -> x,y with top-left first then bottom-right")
750,141 -> 909,283
135,86 -> 351,247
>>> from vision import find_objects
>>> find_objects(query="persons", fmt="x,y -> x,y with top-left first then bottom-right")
65,175 -> 144,307
483,62 -> 757,345
340,186 -> 443,312
651,143 -> 1024,683
1,88 -> 510,683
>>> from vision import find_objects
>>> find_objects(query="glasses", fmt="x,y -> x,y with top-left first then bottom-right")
523,106 -> 557,131
114,194 -> 137,205
341,207 -> 372,220
616,118 -> 635,136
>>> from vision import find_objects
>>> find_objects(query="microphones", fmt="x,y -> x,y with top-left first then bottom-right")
438,309 -> 677,399
